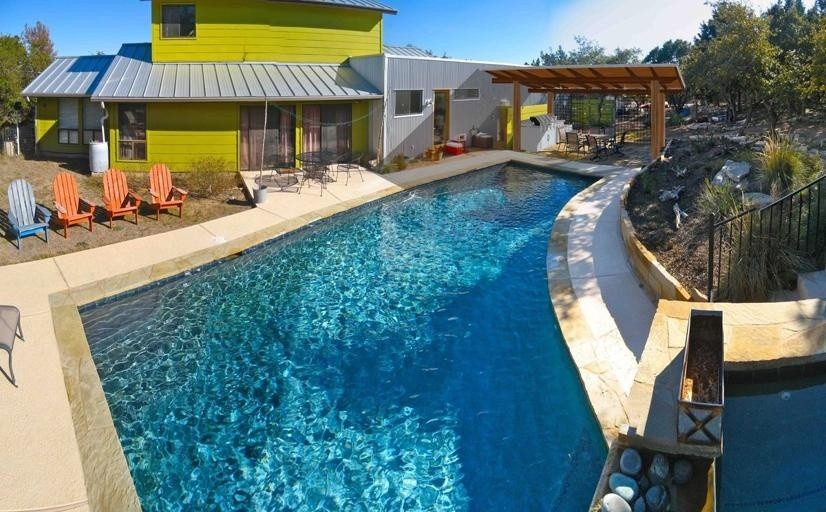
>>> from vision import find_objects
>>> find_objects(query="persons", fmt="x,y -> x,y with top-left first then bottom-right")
623,95 -> 639,114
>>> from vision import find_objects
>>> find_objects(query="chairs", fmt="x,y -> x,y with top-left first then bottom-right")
53,170 -> 96,240
101,168 -> 144,229
147,161 -> 188,222
556,125 -> 630,163
255,147 -> 366,198
5,178 -> 52,249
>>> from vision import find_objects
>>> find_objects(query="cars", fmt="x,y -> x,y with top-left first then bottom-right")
620,101 -> 734,123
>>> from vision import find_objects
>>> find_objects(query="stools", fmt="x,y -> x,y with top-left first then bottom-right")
0,304 -> 25,389
